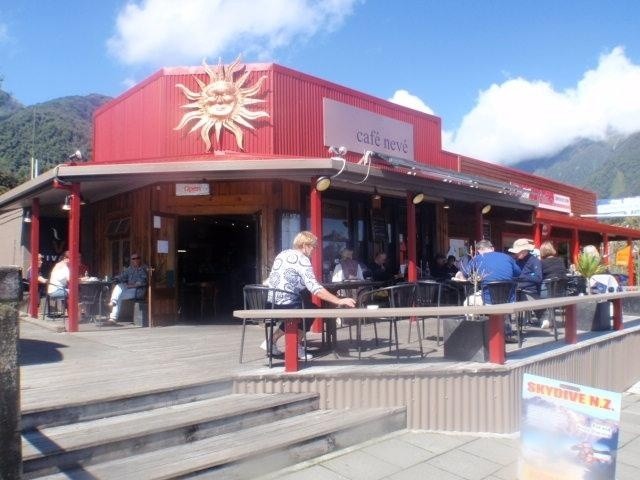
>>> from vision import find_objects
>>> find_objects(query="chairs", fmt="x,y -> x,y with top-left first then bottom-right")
22,272 -> 149,330
190,280 -> 216,321
359,283 -> 423,362
243,284 -> 307,371
450,272 -> 582,349
408,282 -> 457,347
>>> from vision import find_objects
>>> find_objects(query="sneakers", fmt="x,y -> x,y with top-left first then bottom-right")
506,335 -> 519,342
260,334 -> 313,360
541,320 -> 554,329
335,318 -> 342,328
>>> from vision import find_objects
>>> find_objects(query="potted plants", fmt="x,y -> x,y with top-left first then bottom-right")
443,259 -> 490,363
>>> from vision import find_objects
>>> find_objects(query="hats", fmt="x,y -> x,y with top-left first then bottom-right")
508,238 -> 535,254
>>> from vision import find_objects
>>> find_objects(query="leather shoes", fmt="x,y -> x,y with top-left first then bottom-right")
104,304 -> 113,313
103,319 -> 116,325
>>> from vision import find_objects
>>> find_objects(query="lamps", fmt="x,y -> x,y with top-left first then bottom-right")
481,204 -> 491,215
69,150 -> 83,163
24,210 -> 32,223
315,175 -> 331,192
412,190 -> 424,205
61,196 -> 71,211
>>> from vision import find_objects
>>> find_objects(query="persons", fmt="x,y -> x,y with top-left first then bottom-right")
331,237 -> 566,335
259,230 -> 356,360
26,250 -> 149,327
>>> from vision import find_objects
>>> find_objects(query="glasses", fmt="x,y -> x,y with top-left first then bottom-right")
132,257 -> 141,260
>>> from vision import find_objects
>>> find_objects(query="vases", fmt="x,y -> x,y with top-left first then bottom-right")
575,301 -> 596,332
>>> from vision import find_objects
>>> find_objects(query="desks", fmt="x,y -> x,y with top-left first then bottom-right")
298,282 -> 369,358
